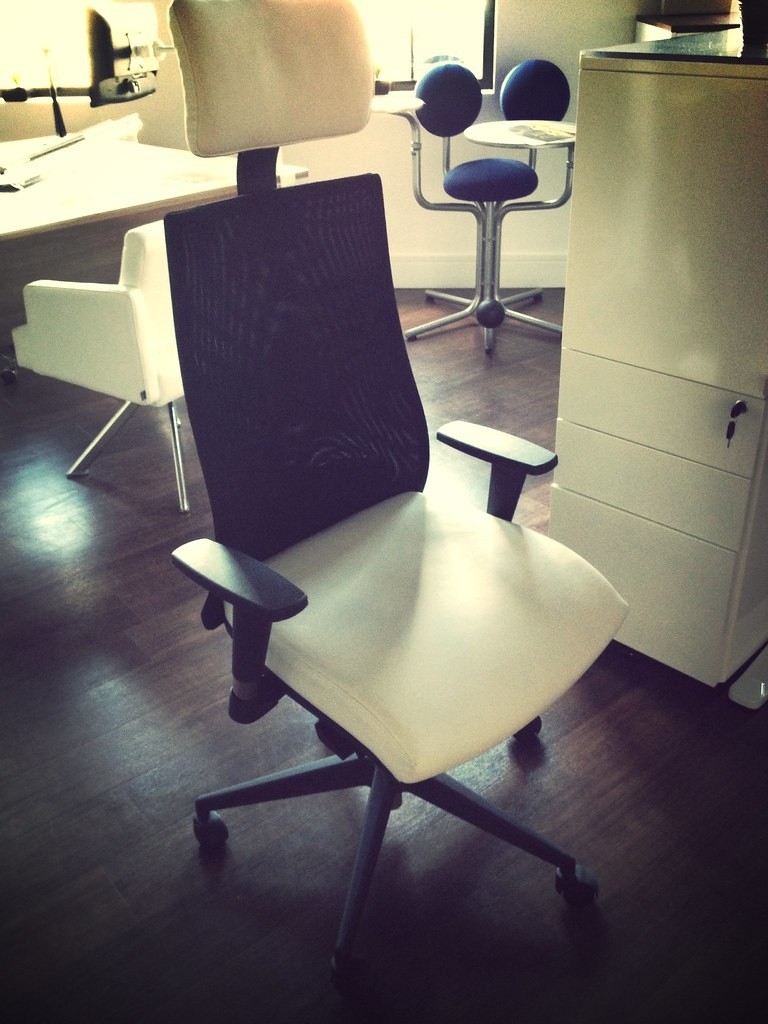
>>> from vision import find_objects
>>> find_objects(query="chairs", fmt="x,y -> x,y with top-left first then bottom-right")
165,1 -> 630,977
12,220 -> 190,514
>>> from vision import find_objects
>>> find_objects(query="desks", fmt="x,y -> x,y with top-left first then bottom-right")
0,132 -> 309,354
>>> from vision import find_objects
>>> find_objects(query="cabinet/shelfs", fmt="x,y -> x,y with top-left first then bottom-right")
547,13 -> 768,709
